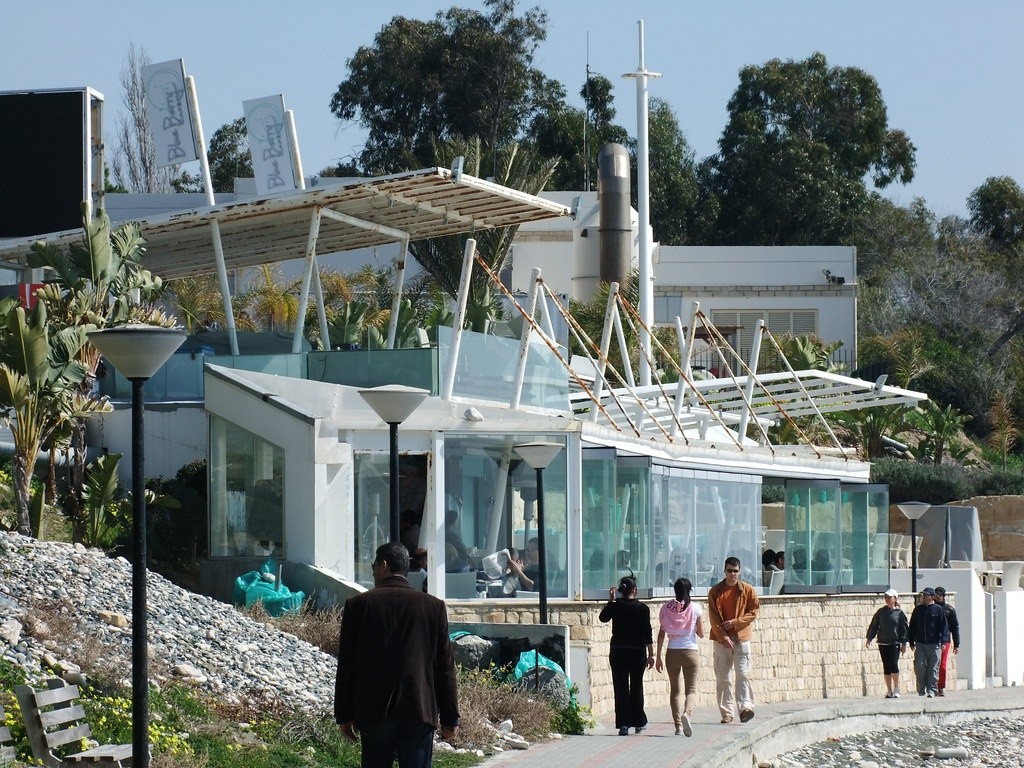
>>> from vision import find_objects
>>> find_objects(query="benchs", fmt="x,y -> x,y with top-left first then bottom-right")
15,678 -> 154,768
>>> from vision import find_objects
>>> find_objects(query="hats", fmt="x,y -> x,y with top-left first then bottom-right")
920,588 -> 935,596
884,589 -> 898,598
935,586 -> 945,595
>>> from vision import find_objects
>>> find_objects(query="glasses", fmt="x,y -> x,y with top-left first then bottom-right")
725,568 -> 739,573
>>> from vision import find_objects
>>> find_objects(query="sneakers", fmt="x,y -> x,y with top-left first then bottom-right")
885,689 -> 900,698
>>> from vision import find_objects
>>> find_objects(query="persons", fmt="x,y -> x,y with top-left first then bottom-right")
334,542 -> 461,768
909,587 -> 960,698
709,557 -> 760,723
656,578 -> 704,737
599,577 -> 655,735
389,506 -> 834,593
866,588 -> 908,698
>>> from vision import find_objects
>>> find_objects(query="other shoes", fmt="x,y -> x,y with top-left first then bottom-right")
681,713 -> 693,737
635,724 -> 647,733
937,687 -> 944,696
721,715 -> 733,723
675,728 -> 681,735
918,691 -> 925,696
927,692 -> 934,698
618,726 -> 628,735
740,709 -> 754,722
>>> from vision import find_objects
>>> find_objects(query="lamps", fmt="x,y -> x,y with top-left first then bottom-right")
822,269 -> 833,283
862,373 -> 889,398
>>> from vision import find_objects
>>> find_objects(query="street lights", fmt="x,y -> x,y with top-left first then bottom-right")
355,383 -> 428,549
895,499 -> 933,593
89,320 -> 192,768
514,439 -> 566,629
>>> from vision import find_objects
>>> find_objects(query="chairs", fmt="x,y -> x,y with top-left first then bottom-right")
406,572 -> 423,593
445,571 -> 477,599
586,521 -> 924,595
514,570 -> 557,597
